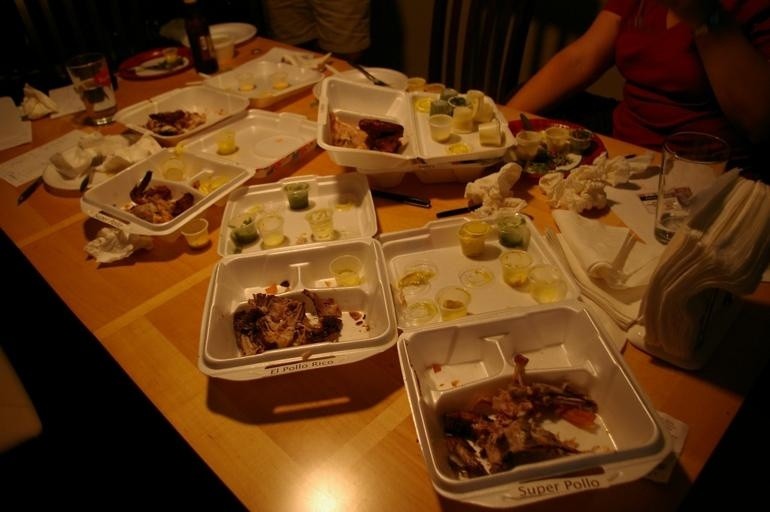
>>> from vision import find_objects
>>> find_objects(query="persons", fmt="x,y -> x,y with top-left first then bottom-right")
265,0 -> 373,67
503,2 -> 770,171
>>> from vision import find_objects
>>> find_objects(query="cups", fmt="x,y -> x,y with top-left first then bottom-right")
396,206 -> 564,328
64,53 -> 119,126
164,129 -> 369,288
653,132 -> 731,245
201,34 -> 235,70
237,66 -> 292,91
407,77 -> 593,160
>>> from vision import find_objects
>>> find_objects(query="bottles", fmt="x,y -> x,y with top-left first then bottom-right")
183,0 -> 218,76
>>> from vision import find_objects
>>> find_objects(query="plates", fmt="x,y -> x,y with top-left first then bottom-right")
117,46 -> 193,82
188,21 -> 257,50
507,117 -> 609,184
40,130 -> 163,192
312,67 -> 407,103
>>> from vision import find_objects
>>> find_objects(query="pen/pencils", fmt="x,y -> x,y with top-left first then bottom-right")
18,176 -> 43,203
371,190 -> 431,208
437,204 -> 485,220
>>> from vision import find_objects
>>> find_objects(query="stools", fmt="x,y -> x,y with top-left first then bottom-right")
0,352 -> 43,451
